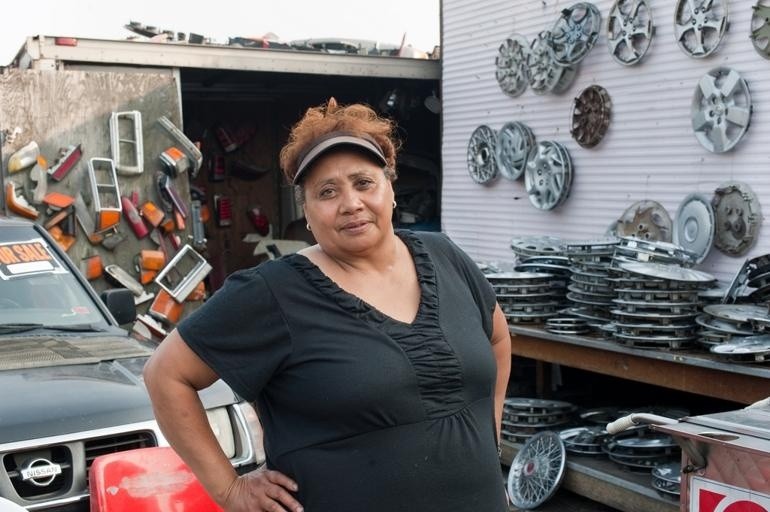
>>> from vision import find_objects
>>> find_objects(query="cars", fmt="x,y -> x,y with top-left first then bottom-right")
0,217 -> 266,511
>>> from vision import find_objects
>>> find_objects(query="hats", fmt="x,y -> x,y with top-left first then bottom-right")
291,130 -> 387,185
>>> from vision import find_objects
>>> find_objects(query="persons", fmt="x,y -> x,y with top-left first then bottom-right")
143,96 -> 513,512
